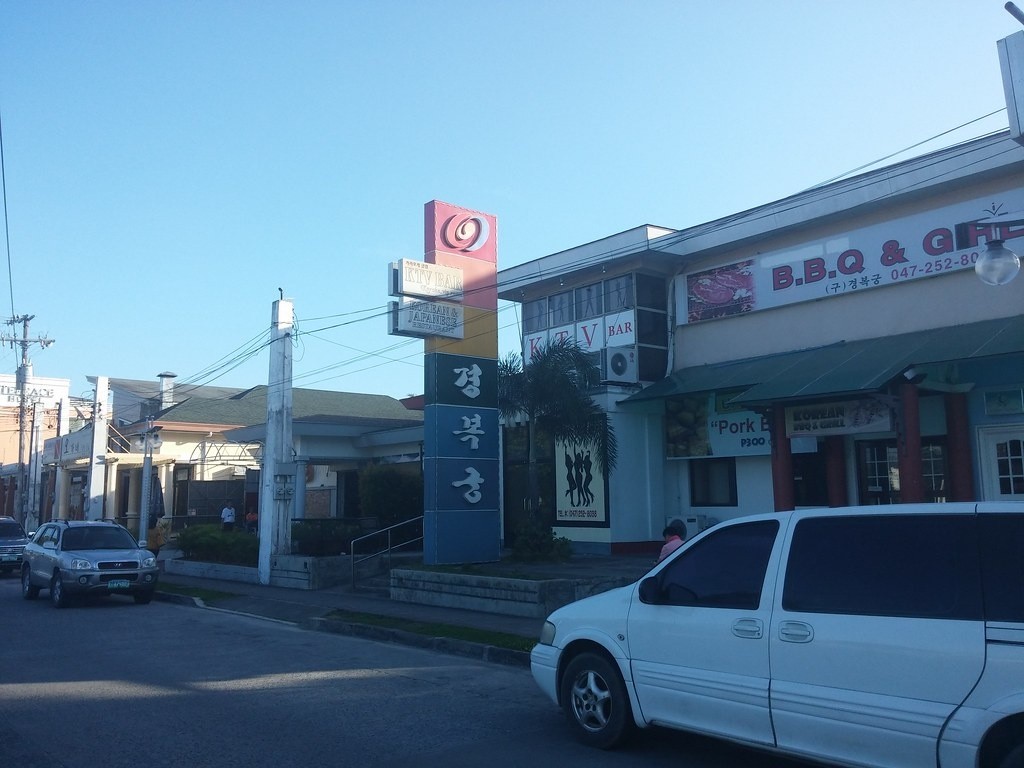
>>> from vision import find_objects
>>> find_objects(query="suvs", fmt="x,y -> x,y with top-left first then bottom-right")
20,515 -> 160,605
0,515 -> 37,578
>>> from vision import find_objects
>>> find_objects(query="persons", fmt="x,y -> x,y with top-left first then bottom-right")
220,499 -> 237,532
244,506 -> 257,523
525,273 -> 633,331
561,441 -> 597,508
650,526 -> 687,567
147,516 -> 161,559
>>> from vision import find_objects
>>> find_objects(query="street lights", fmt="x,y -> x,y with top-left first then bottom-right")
131,412 -> 163,549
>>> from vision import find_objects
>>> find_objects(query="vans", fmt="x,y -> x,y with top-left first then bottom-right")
523,497 -> 1024,768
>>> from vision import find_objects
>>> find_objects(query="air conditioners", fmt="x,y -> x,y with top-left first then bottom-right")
666,515 -> 703,540
601,348 -> 637,384
